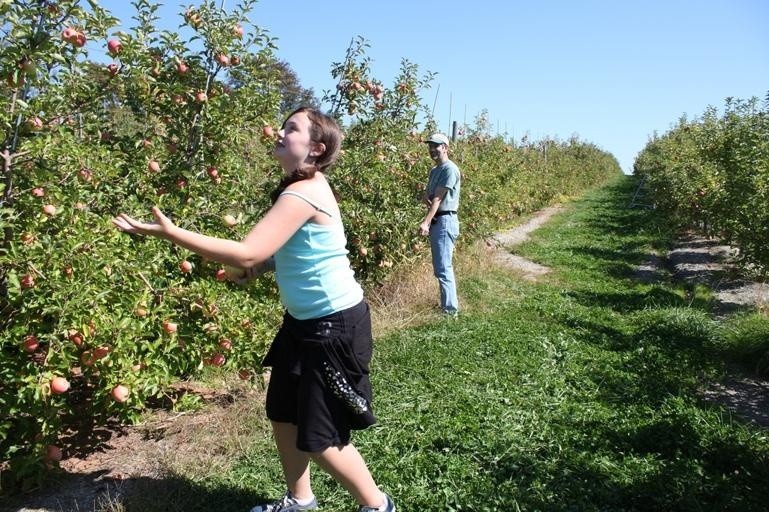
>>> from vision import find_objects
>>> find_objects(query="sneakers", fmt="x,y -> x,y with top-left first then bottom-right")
250,494 -> 317,512
357,493 -> 395,512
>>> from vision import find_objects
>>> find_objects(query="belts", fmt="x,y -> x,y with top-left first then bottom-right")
436,211 -> 457,215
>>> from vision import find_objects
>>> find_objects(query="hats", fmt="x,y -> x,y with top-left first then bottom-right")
423,134 -> 449,146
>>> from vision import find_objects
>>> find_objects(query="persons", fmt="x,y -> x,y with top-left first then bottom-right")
418,133 -> 461,322
111,107 -> 396,512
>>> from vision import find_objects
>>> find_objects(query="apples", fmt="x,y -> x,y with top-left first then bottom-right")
21,14 -> 425,464
